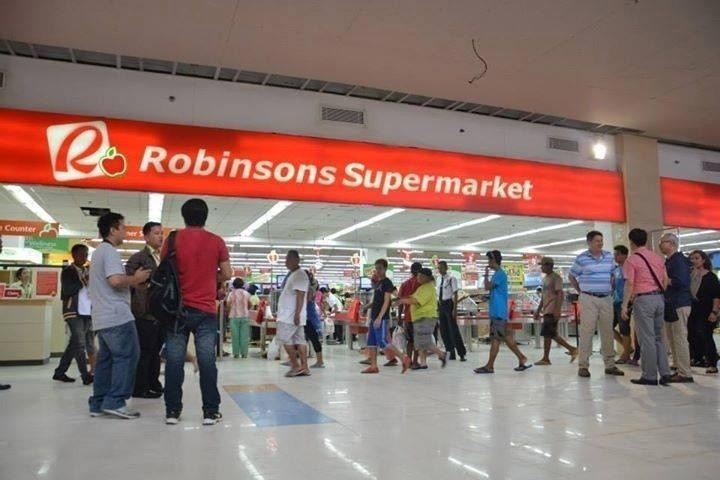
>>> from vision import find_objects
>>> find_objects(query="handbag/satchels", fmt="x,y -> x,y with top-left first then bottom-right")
664,296 -> 679,322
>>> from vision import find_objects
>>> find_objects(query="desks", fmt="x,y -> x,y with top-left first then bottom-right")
0,295 -> 59,368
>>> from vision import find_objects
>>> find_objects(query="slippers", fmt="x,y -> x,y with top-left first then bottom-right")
284,368 -> 307,377
569,348 -> 578,363
473,367 -> 493,373
310,364 -> 324,368
514,364 -> 532,371
534,360 -> 552,364
300,372 -> 311,375
279,362 -> 291,366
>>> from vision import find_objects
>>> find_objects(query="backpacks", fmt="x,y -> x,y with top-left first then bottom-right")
677,252 -> 702,300
143,231 -> 189,338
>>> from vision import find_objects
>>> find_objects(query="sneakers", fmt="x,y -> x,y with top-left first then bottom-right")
166,411 -> 180,424
89,412 -> 121,417
202,412 -> 223,425
100,405 -> 140,419
359,352 -> 446,373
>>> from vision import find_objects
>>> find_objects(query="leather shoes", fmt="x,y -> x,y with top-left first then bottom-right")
605,366 -> 624,375
460,354 -> 467,361
449,349 -> 455,359
671,375 -> 693,382
578,368 -> 590,377
53,373 -> 75,382
84,375 -> 94,385
631,377 -> 658,385
706,368 -> 718,373
132,388 -> 162,398
659,375 -> 672,384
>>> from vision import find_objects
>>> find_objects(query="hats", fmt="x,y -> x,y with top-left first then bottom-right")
537,257 -> 554,265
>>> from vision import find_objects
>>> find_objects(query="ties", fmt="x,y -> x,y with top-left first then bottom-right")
439,277 -> 443,306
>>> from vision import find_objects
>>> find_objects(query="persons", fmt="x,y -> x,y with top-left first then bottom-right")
9,268 -> 33,299
53,197 -> 232,424
226,250 -> 578,374
567,228 -> 720,386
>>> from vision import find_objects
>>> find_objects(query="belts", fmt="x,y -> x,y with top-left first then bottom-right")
638,291 -> 661,296
582,291 -> 609,297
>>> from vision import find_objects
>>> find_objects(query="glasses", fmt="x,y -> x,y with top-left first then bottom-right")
658,240 -> 671,245
